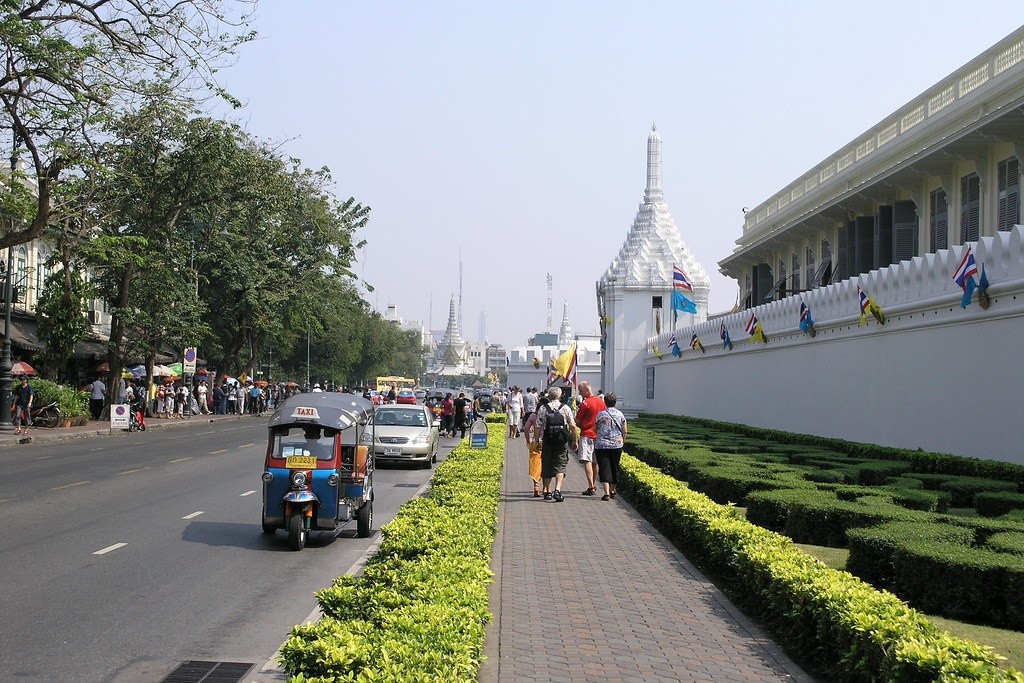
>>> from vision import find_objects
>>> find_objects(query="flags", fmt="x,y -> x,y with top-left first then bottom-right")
667,334 -> 680,356
670,289 -> 697,322
689,332 -> 699,350
953,244 -> 988,308
673,264 -> 694,294
652,347 -> 662,356
796,300 -> 815,336
720,323 -> 730,350
546,342 -> 576,389
856,284 -> 884,327
745,312 -> 768,344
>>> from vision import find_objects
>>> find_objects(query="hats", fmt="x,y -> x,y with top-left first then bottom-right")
314,382 -> 321,388
20,375 -> 30,380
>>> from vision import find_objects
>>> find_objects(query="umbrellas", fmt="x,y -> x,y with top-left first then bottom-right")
97,356 -> 207,382
9,356 -> 39,375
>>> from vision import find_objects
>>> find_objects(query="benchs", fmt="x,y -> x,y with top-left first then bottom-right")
279,442 -> 368,484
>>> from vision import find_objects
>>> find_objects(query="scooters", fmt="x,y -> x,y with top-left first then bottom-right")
129,401 -> 148,432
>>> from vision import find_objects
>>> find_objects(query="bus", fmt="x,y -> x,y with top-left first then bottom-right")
377,375 -> 417,394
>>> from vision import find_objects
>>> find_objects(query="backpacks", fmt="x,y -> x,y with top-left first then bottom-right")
541,403 -> 570,448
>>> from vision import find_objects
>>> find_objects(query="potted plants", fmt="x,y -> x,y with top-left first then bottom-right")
58,387 -> 92,427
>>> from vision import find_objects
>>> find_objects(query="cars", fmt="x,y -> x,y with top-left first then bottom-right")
358,404 -> 442,470
416,391 -> 426,399
397,390 -> 416,404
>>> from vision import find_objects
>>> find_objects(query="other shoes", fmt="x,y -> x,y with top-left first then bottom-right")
581,486 -> 597,495
175,416 -> 180,419
601,495 -> 610,501
92,416 -> 96,419
207,411 -> 214,415
610,493 -> 616,499
158,416 -> 164,419
15,430 -> 22,435
461,435 -> 464,438
443,432 -> 445,437
166,417 -> 170,419
447,434 -> 449,438
534,488 -> 540,497
516,432 -> 520,438
170,417 -> 174,419
180,417 -> 185,419
544,492 -> 553,500
23,430 -> 28,436
95,418 -> 101,421
235,412 -> 238,415
553,490 -> 565,502
452,434 -> 455,438
196,412 -> 202,415
228,412 -> 231,415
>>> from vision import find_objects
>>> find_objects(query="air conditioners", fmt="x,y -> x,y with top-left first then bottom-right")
88,311 -> 101,324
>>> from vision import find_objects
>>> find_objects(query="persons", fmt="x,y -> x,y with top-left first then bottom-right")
387,385 -> 398,404
86,376 -> 379,420
439,390 -> 484,438
12,374 -> 36,435
379,391 -> 386,404
489,380 -> 629,502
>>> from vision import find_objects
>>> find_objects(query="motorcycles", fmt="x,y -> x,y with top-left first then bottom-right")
7,394 -> 62,428
259,391 -> 378,550
426,387 -> 509,431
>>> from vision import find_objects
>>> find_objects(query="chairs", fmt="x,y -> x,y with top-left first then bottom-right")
382,413 -> 395,421
